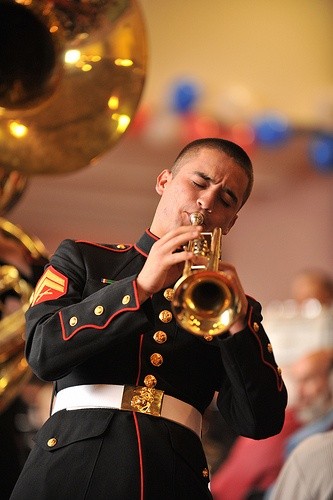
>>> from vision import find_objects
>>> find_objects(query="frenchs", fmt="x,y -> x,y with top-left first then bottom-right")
0,0 -> 149,408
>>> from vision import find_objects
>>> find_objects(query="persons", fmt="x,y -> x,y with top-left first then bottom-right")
204,269 -> 333,500
8,137 -> 289,500
274,344 -> 333,496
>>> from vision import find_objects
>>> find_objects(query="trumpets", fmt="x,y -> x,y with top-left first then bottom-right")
172,211 -> 244,341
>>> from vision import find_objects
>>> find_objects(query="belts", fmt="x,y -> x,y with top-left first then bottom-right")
50,384 -> 202,441
